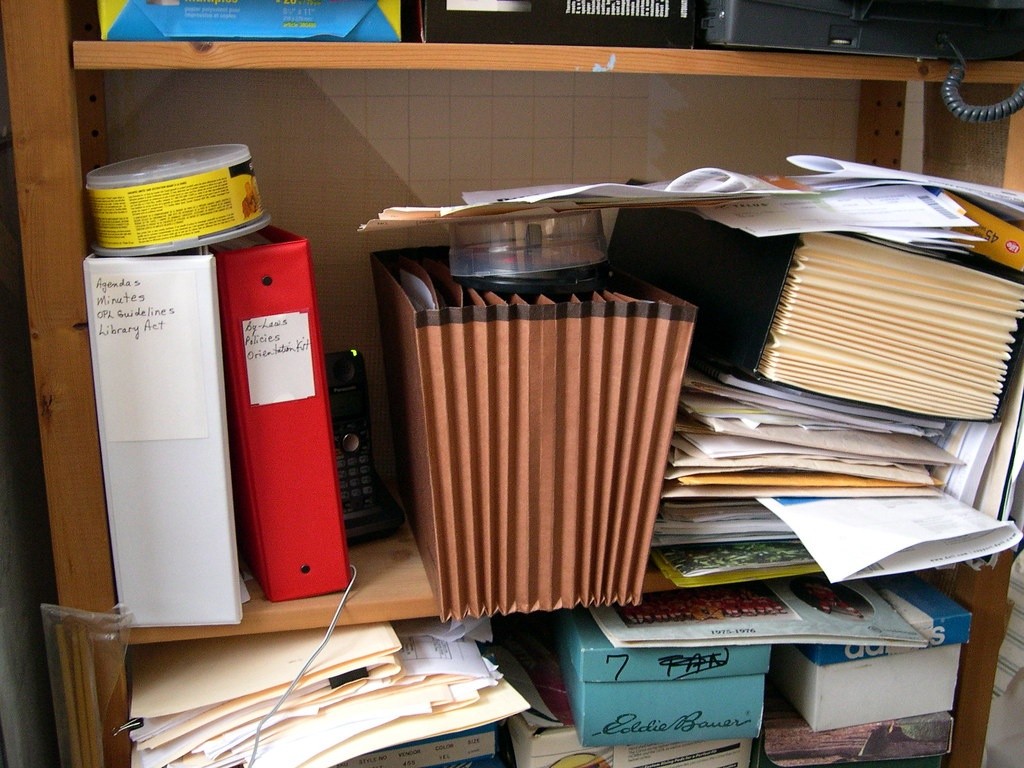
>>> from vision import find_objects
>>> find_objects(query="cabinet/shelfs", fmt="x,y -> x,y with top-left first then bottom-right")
0,1 -> 1024,768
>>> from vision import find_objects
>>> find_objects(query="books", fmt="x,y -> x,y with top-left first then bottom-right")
649,354 -> 1024,589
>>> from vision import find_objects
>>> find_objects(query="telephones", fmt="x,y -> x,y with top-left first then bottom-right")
326,349 -> 406,546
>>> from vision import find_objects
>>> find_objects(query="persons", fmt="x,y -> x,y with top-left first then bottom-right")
620,592 -> 788,625
804,580 -> 863,620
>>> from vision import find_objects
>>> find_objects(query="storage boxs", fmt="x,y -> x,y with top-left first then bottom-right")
330,721 -> 496,768
771,582 -> 974,733
550,609 -> 772,746
98,0 -> 401,44
480,617 -> 750,768
